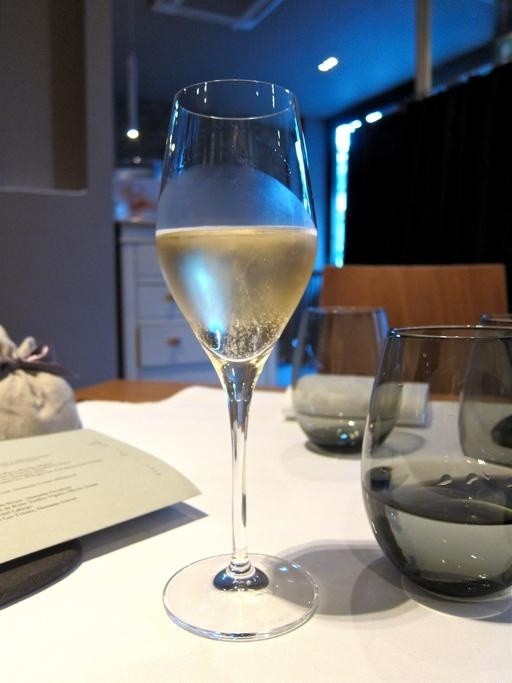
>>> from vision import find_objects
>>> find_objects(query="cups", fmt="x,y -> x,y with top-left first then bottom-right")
360,323 -> 511,605
458,313 -> 511,466
293,305 -> 402,455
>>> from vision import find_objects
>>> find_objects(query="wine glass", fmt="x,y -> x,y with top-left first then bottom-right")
154,75 -> 322,644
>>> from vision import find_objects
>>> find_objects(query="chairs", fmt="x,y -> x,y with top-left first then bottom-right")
315,261 -> 511,397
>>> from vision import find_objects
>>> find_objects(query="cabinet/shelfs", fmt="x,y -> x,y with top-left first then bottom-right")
113,224 -> 279,382
